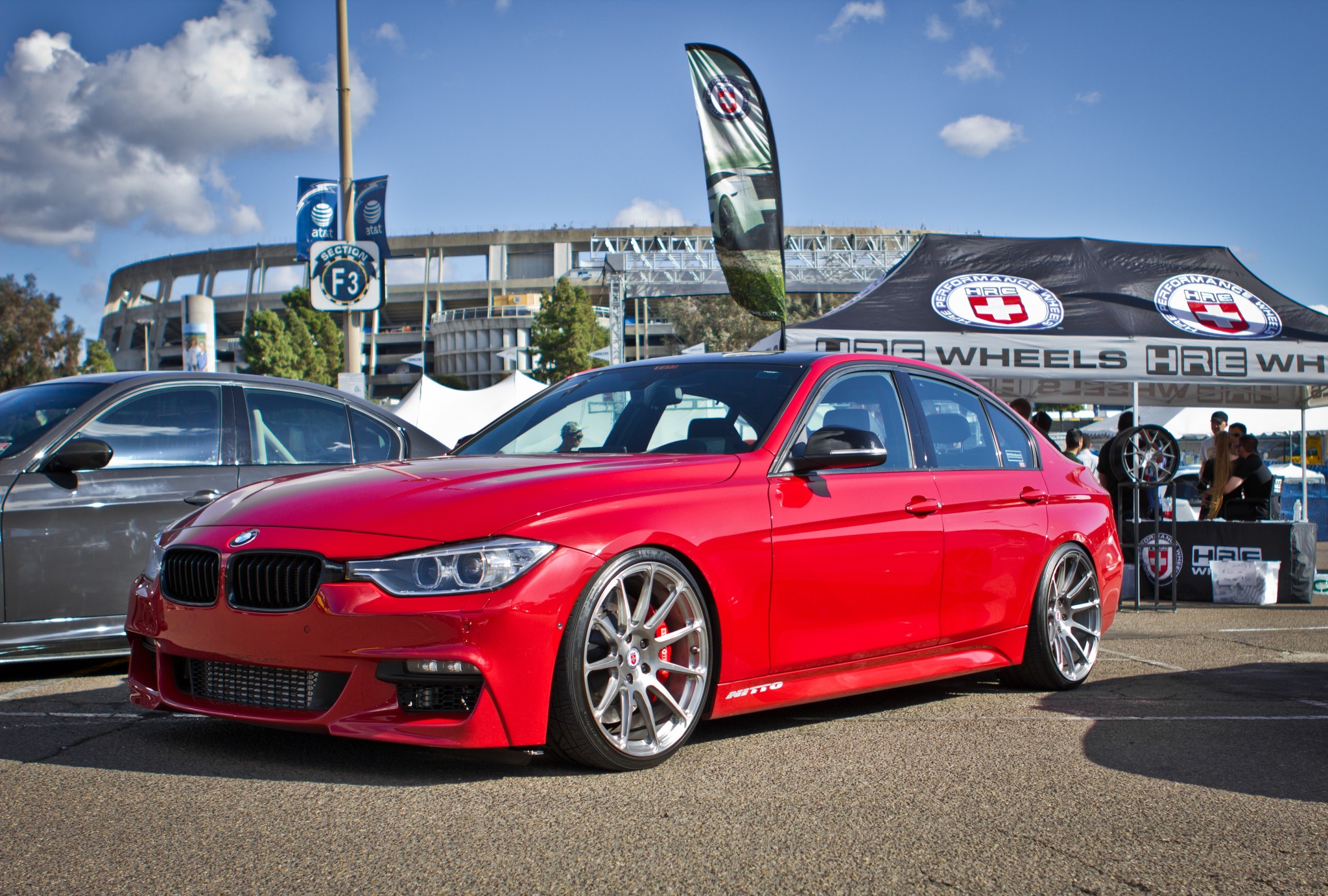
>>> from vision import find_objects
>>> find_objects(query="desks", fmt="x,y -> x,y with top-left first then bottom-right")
1125,518 -> 1318,605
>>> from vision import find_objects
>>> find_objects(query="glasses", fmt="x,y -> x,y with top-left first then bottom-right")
1231,433 -> 1244,437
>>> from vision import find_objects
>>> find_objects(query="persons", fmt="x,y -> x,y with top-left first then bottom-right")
1010,398 -> 1052,436
553,421 -> 587,452
1096,411 -> 1160,528
183,338 -> 207,372
1061,428 -> 1100,484
1198,411 -> 1280,521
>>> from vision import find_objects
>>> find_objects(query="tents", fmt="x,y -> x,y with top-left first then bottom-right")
747,232 -> 1328,525
1081,304 -> 1328,466
389,370 -> 758,455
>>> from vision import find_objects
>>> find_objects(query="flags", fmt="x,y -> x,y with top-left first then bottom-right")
684,41 -> 786,322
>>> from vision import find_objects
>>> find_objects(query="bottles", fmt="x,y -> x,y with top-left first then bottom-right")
1293,499 -> 1302,521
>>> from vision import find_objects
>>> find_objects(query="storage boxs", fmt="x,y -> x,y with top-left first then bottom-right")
1206,557 -> 1283,606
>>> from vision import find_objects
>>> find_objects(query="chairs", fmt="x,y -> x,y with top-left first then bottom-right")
641,407 -> 1016,468
254,416 -> 384,466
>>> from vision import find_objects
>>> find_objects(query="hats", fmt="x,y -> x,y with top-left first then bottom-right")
1212,411 -> 1228,423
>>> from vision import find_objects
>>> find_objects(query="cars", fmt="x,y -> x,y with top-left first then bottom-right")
122,349 -> 1125,774
0,369 -> 453,666
